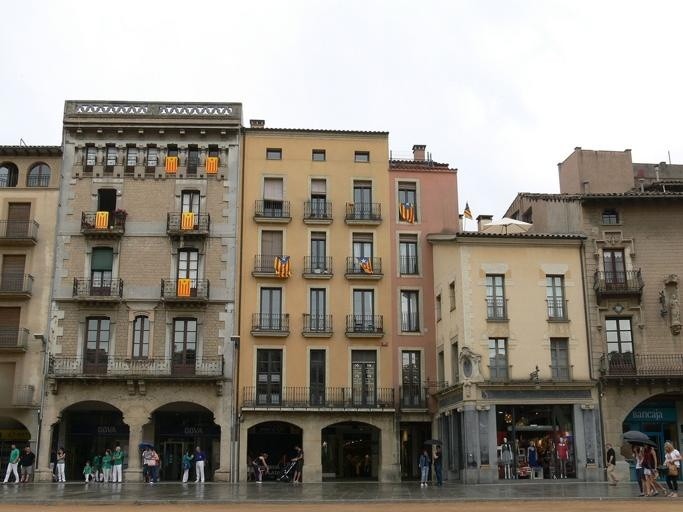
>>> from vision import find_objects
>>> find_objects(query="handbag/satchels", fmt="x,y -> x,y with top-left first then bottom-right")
667,461 -> 679,477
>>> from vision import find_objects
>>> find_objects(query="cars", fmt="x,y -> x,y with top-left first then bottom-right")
494,443 -> 574,477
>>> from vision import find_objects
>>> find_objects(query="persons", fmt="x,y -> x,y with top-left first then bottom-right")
252,453 -> 269,484
0,443 -> 207,484
606,443 -> 619,485
500,438 -> 513,479
433,446 -> 443,486
669,294 -> 680,326
632,442 -> 683,498
526,441 -> 538,468
290,443 -> 304,483
556,436 -> 569,479
419,447 -> 431,487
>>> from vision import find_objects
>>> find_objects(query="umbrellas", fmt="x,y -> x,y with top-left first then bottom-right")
356,257 -> 373,274
627,439 -> 658,448
481,217 -> 533,235
138,441 -> 154,449
423,439 -> 442,445
400,202 -> 415,224
620,431 -> 650,441
274,255 -> 291,278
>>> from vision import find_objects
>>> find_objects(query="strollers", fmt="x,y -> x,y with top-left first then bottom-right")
275,459 -> 297,483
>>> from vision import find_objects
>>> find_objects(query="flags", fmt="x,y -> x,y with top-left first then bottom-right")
464,203 -> 472,219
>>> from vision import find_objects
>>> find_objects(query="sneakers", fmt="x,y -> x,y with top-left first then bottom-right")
640,490 -> 679,498
